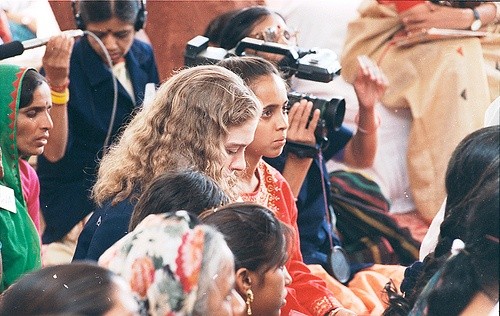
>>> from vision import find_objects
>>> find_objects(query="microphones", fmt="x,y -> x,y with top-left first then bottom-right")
0,29 -> 84,61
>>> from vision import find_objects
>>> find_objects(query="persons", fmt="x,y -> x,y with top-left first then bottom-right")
0,0 -> 500,316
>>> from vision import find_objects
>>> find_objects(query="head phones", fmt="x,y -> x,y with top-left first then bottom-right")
72,0 -> 147,31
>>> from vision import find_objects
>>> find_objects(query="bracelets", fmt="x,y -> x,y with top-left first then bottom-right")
46,76 -> 70,105
355,112 -> 381,134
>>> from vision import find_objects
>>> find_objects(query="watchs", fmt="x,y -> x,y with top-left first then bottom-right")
470,8 -> 481,31
